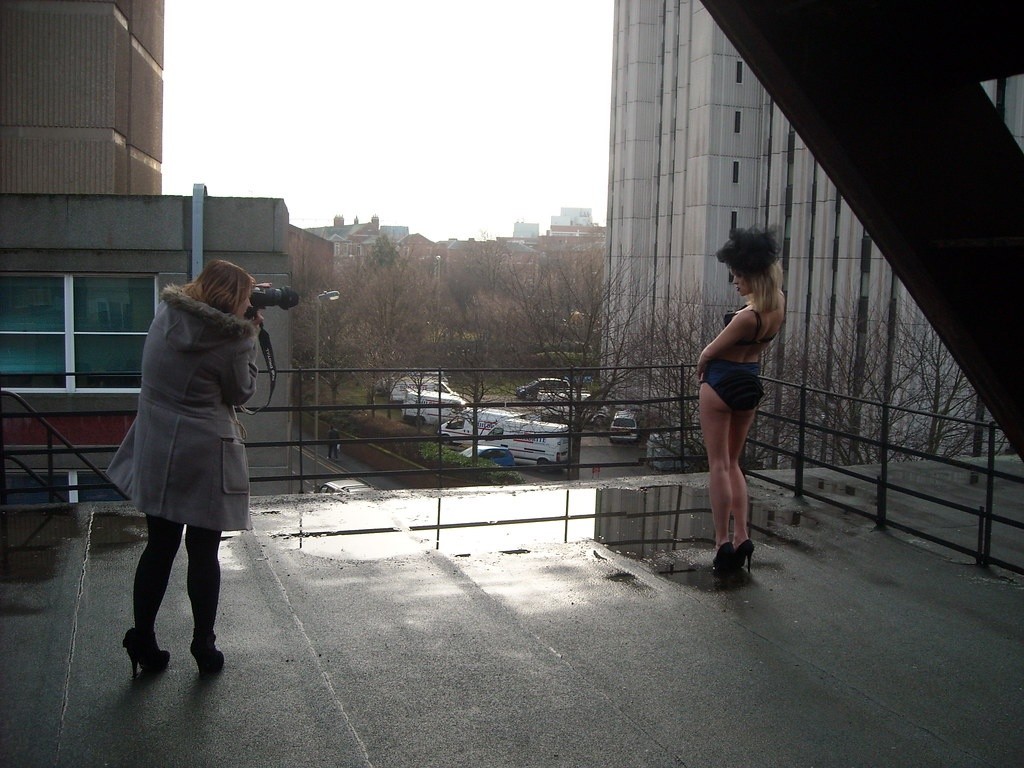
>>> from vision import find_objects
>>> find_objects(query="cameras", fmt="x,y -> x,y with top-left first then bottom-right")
244,286 -> 299,320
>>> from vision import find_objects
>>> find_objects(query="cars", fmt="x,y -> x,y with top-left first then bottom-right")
458,446 -> 516,472
609,410 -> 638,445
515,378 -> 570,401
306,479 -> 374,492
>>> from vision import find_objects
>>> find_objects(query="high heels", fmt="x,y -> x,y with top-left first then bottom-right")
191,628 -> 224,676
123,628 -> 170,678
712,542 -> 734,578
734,539 -> 754,573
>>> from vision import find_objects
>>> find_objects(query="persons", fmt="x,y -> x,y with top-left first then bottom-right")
696,229 -> 786,580
105,260 -> 261,679
326,424 -> 340,460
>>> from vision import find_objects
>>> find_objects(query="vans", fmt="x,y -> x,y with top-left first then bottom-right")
401,386 -> 466,427
390,377 -> 454,406
437,404 -> 525,445
478,419 -> 568,472
537,390 -> 610,427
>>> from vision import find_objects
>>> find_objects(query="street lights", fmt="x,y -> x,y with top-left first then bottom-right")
314,291 -> 340,492
